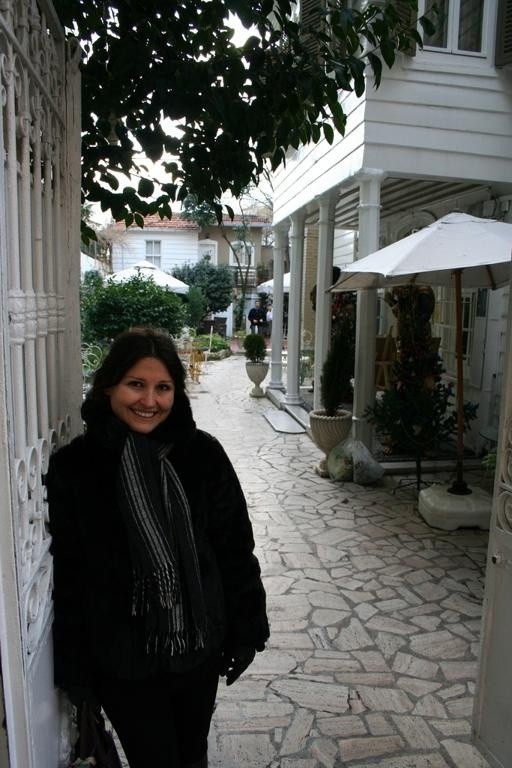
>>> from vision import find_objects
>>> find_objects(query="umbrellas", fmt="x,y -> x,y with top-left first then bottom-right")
103,260 -> 190,295
323,212 -> 512,496
257,271 -> 290,294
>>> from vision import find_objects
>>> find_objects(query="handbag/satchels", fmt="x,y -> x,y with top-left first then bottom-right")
68,700 -> 122,768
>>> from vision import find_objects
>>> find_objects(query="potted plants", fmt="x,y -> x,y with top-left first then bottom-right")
309,340 -> 355,471
242,333 -> 270,397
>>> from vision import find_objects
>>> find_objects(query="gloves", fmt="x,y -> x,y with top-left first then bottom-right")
218,642 -> 256,686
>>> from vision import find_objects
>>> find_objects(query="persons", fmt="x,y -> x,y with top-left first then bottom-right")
46,328 -> 270,768
248,301 -> 267,333
266,304 -> 273,337
